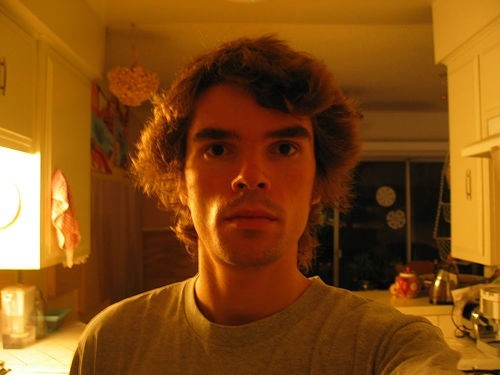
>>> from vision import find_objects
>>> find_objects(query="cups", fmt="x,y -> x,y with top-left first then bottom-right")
480,287 -> 500,342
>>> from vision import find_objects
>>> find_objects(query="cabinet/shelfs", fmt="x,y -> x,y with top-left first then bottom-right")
439,18 -> 500,266
0,9 -> 91,270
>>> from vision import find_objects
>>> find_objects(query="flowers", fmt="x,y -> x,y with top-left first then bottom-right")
108,45 -> 159,105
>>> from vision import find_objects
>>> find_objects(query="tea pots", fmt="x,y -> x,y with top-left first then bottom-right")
390,267 -> 422,298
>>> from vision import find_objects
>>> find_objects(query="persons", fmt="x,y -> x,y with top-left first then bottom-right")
70,33 -> 464,375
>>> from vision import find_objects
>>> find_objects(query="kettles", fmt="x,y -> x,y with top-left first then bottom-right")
429,263 -> 458,304
